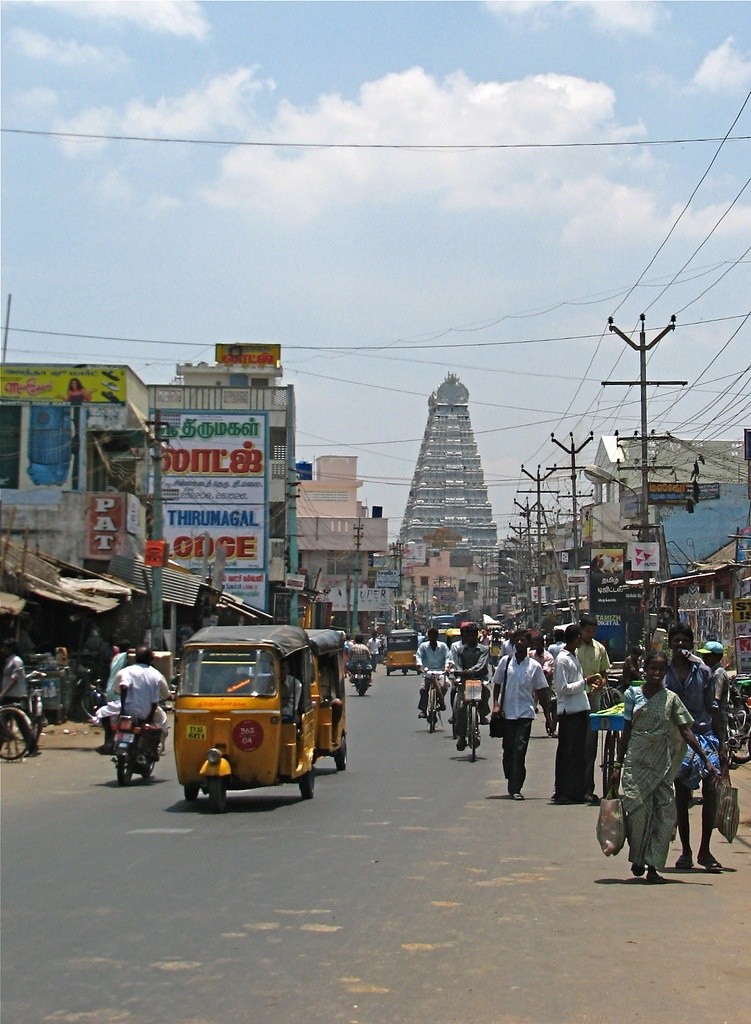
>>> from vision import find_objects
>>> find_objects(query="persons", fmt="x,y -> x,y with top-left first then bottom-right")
317,653 -> 343,746
622,646 -> 643,692
256,653 -> 302,720
101,643 -> 164,745
609,650 -> 719,883
696,641 -> 731,786
581,614 -> 613,802
56,379 -> 96,405
478,628 -> 567,738
415,628 -> 450,718
344,632 -> 386,696
0,638 -> 40,757
450,624 -> 490,751
96,646 -> 168,754
552,625 -> 607,805
660,623 -> 729,872
491,630 -> 554,800
447,626 -> 468,724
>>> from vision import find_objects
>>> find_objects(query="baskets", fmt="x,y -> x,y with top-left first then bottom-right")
463,682 -> 482,700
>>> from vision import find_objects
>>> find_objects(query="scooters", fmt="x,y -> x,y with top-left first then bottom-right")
348,657 -> 373,697
725,672 -> 751,771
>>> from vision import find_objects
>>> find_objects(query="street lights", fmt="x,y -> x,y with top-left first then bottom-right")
510,535 -> 543,609
583,463 -> 652,653
506,557 -> 535,629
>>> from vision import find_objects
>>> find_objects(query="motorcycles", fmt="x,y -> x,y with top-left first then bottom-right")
169,624 -> 322,814
418,614 -> 507,667
303,628 -> 349,771
385,627 -> 422,676
110,696 -> 173,787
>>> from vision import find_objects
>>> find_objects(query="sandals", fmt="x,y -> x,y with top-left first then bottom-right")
511,792 -> 524,800
631,864 -> 645,876
646,873 -> 665,884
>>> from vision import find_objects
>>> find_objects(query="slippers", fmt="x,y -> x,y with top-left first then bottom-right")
675,856 -> 694,868
697,854 -> 722,872
24,748 -> 41,757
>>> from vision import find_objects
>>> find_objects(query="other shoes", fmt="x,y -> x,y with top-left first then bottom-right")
457,738 -> 467,751
439,706 -> 446,711
96,748 -> 113,755
99,743 -> 115,750
449,716 -> 453,723
418,712 -> 427,718
480,716 -> 488,725
585,794 -> 599,801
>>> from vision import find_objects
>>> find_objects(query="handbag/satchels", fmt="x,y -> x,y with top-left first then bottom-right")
710,777 -> 739,843
596,784 -> 625,856
490,710 -> 505,738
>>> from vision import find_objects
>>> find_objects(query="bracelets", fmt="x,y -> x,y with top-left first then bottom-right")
614,763 -> 621,767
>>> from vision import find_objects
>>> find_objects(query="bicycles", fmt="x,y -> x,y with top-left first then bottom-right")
0,701 -> 37,764
600,682 -> 624,800
446,669 -> 488,763
419,666 -> 447,733
75,666 -> 108,728
20,663 -> 56,744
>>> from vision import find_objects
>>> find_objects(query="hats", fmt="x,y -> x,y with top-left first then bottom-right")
696,641 -> 723,654
